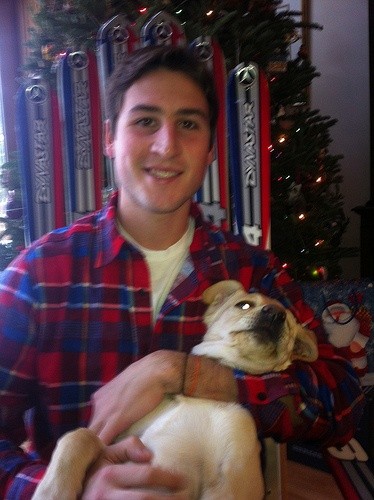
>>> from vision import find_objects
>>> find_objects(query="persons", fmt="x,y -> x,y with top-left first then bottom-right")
1,44 -> 365,499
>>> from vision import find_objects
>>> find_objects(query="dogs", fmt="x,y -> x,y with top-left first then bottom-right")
30,279 -> 320,499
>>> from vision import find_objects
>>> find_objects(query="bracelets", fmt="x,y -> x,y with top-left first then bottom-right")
187,355 -> 200,396
180,351 -> 188,390
